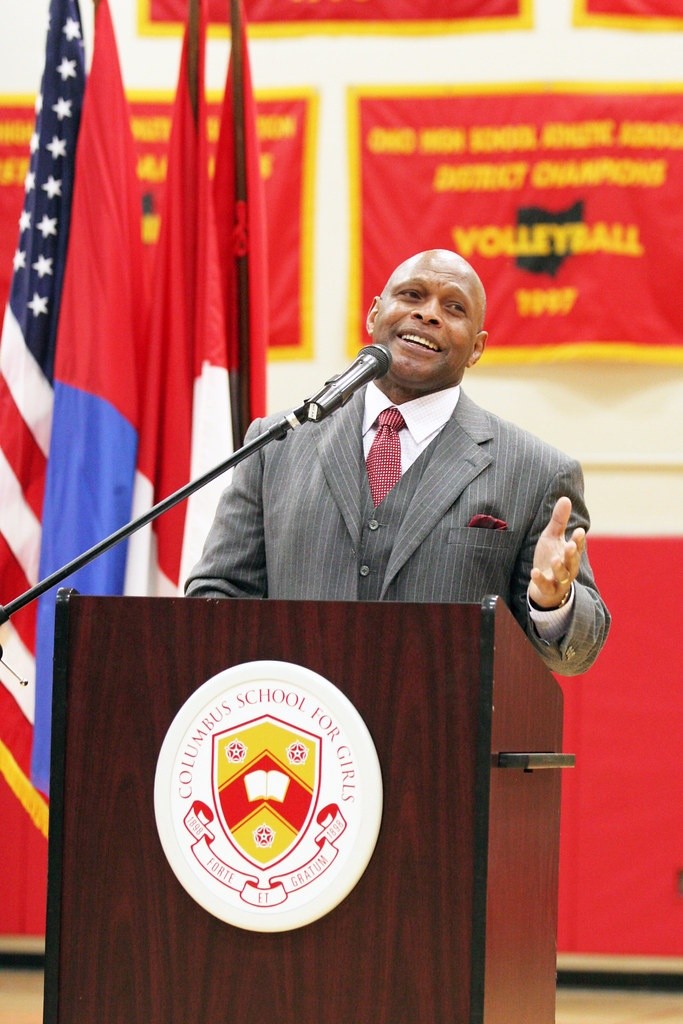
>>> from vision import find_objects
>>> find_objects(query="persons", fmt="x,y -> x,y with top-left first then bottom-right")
183,249 -> 611,676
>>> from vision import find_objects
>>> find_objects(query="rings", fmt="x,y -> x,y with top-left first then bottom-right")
556,571 -> 571,584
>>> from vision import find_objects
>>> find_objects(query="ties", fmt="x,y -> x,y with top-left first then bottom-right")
366,407 -> 407,508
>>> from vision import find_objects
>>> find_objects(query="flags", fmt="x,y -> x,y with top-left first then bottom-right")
0,0 -> 683,936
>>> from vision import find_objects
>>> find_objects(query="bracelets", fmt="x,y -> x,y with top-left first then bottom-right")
558,592 -> 571,608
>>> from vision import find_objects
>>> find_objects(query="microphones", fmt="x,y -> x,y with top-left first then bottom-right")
304,343 -> 393,423
